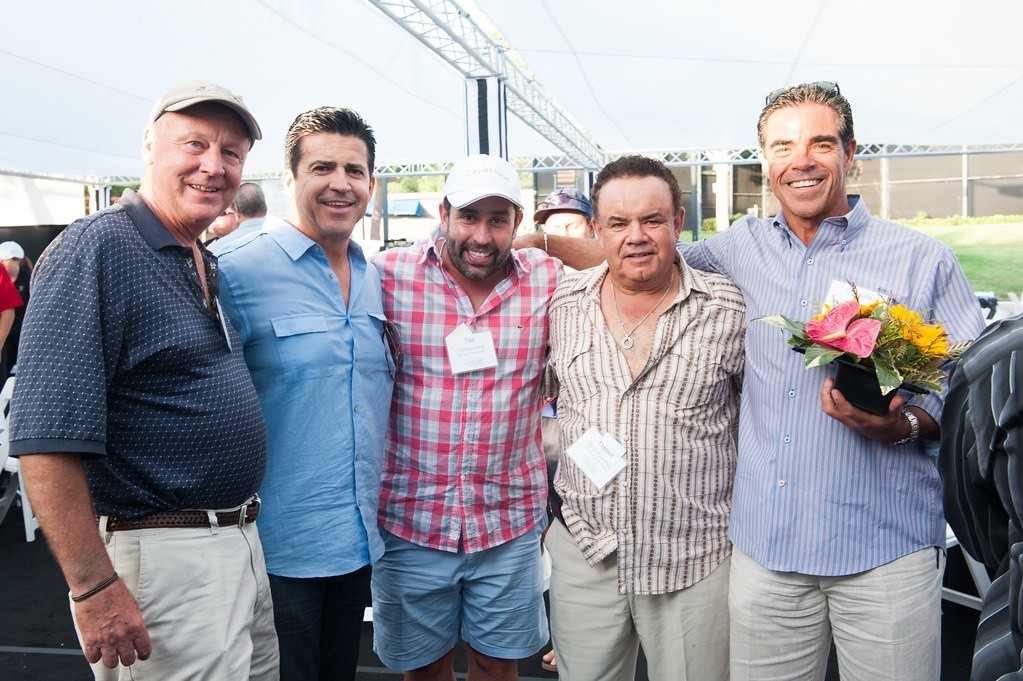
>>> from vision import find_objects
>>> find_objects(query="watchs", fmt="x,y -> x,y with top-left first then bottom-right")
888,409 -> 919,447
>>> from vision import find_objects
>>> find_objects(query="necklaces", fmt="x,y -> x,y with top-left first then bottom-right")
609,268 -> 673,350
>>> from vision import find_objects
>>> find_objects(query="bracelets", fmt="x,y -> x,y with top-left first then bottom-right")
544,231 -> 547,253
71,572 -> 118,602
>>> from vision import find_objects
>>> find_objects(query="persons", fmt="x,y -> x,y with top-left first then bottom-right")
8,86 -> 279,681
513,82 -> 986,681
533,188 -> 594,671
206,107 -> 396,680
368,152 -> 565,680
543,156 -> 745,680
0,260 -> 23,363
0,241 -> 38,473
203,183 -> 267,254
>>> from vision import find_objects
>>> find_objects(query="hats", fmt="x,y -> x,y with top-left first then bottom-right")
533,188 -> 596,220
1,241 -> 25,260
445,153 -> 524,210
151,78 -> 264,148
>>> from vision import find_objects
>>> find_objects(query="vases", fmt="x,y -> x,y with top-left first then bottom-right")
791,347 -> 931,415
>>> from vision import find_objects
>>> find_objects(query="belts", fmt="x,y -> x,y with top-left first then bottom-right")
98,502 -> 259,530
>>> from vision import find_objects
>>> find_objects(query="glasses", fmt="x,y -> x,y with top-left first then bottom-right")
765,80 -> 841,104
536,192 -> 594,209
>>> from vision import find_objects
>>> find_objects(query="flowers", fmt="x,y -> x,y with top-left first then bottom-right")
752,277 -> 973,396
